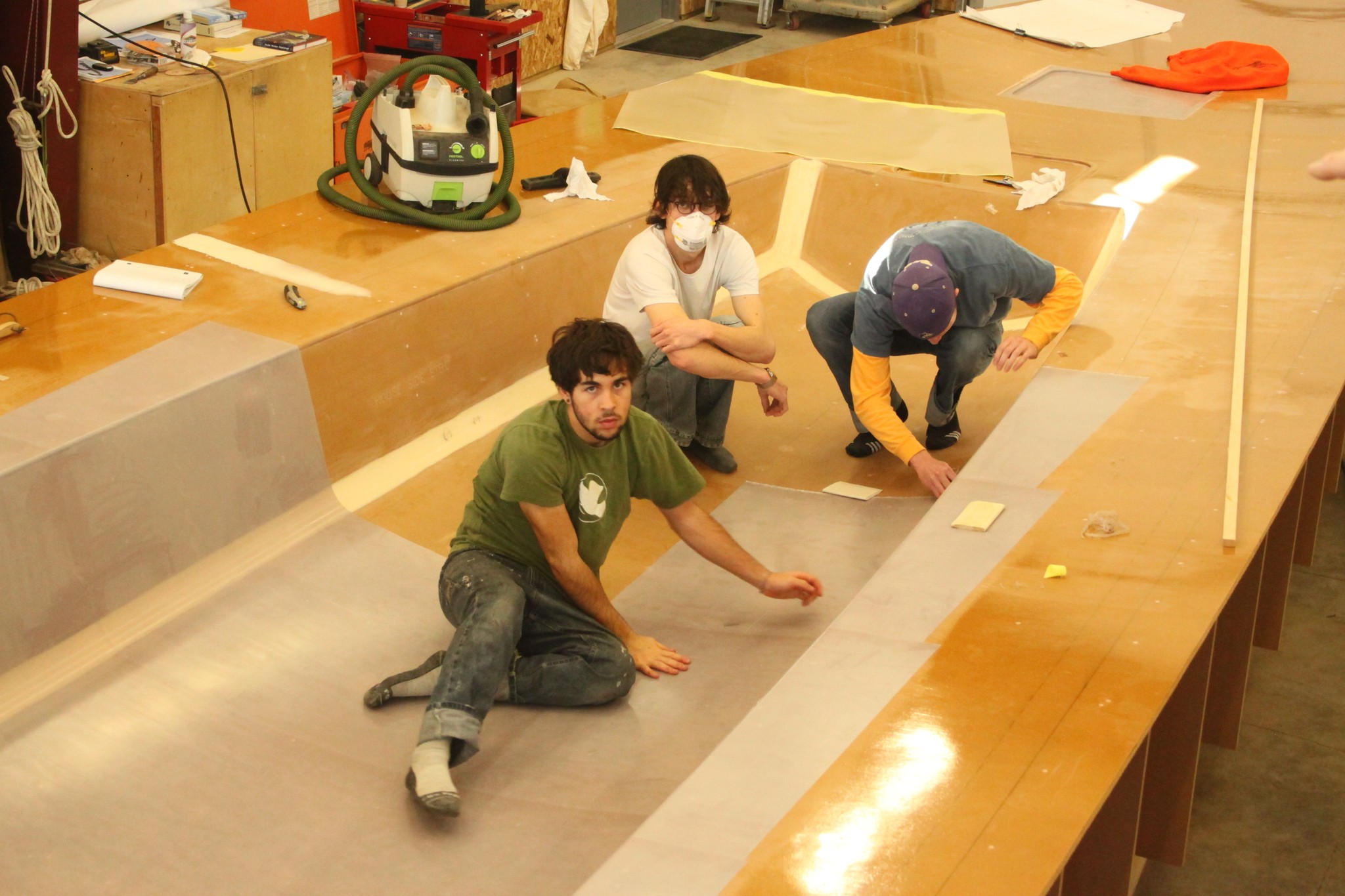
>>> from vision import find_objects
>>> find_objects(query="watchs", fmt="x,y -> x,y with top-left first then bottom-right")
754,368 -> 777,389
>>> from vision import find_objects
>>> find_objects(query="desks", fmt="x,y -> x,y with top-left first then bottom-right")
69,23 -> 336,261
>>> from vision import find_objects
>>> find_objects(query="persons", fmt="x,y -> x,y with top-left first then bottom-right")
807,220 -> 1084,498
364,317 -> 821,816
602,154 -> 788,473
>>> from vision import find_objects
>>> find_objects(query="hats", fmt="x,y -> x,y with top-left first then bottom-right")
891,243 -> 955,340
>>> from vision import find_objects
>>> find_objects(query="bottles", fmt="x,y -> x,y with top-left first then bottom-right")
179,10 -> 197,59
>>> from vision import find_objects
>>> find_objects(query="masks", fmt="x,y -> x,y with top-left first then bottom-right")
671,211 -> 716,253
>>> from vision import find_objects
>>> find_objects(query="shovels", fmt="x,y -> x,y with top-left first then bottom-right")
123,65 -> 159,84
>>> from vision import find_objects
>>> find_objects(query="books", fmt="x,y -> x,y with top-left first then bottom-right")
164,6 -> 247,38
485,9 -> 518,23
253,30 -> 327,52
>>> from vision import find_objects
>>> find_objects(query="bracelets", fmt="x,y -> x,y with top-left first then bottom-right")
759,572 -> 772,593
